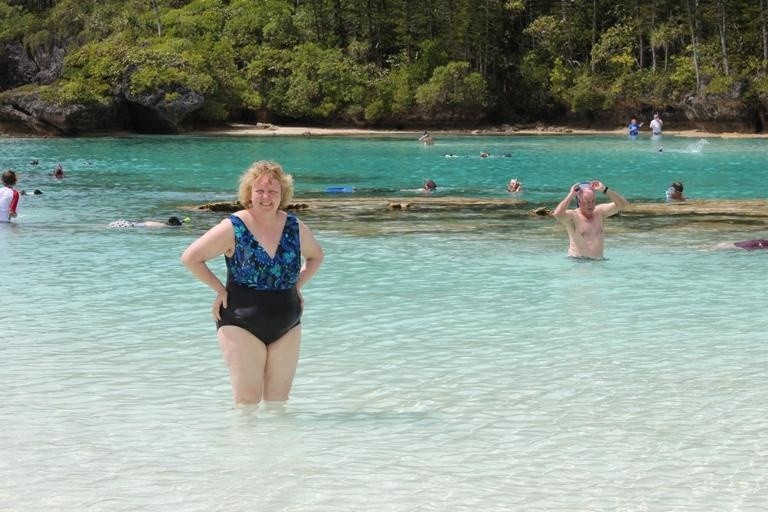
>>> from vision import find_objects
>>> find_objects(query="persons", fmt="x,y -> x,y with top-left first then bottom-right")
650,113 -> 664,136
352,180 -> 436,194
628,118 -> 644,135
181,160 -> 325,408
0,170 -> 20,224
107,216 -> 182,228
53,164 -> 64,179
552,180 -> 628,259
710,239 -> 768,251
666,182 -> 683,200
419,131 -> 433,146
506,179 -> 521,192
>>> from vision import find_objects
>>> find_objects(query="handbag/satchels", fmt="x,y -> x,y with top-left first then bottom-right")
603,187 -> 608,194
